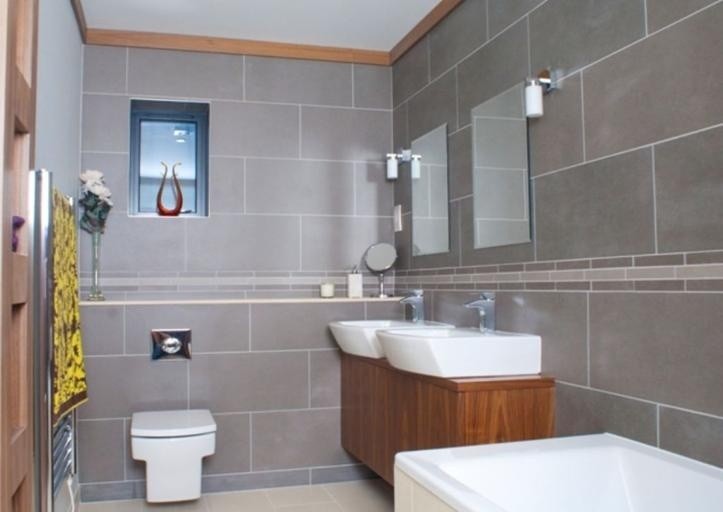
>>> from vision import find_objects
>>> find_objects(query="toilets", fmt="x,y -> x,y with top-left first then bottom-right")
129,408 -> 218,504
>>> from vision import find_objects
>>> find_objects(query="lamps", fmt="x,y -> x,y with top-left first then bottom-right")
409,155 -> 421,178
383,146 -> 411,181
522,68 -> 557,120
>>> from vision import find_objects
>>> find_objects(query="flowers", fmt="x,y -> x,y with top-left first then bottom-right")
74,166 -> 114,237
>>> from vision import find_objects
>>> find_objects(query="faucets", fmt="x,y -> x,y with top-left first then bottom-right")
464,292 -> 496,333
399,288 -> 424,321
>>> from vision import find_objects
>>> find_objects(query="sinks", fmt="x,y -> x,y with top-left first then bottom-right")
326,318 -> 454,360
373,326 -> 542,377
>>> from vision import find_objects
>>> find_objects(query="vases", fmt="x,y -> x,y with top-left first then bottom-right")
86,229 -> 103,301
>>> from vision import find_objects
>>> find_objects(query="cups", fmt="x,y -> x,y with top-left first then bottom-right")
319,271 -> 334,299
347,271 -> 365,300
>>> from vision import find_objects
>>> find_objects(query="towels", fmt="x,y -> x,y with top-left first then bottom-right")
49,190 -> 89,430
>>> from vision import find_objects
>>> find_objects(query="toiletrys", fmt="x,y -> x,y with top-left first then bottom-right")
346,264 -> 363,298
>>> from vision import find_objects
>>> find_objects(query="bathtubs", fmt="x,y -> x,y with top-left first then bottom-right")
393,431 -> 722,512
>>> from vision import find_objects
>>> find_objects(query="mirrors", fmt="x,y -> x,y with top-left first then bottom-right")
468,81 -> 532,250
408,122 -> 451,258
